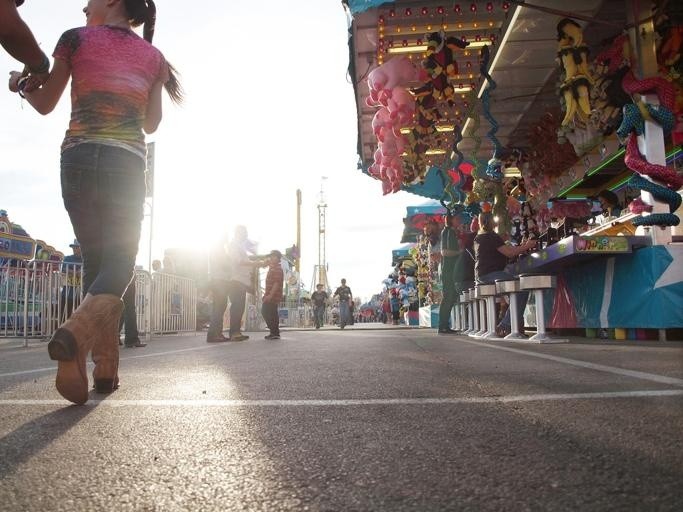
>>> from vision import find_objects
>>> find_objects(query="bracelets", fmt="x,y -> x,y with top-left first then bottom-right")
28,55 -> 49,73
16,76 -> 28,91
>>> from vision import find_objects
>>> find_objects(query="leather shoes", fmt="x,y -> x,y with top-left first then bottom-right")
125,339 -> 147,348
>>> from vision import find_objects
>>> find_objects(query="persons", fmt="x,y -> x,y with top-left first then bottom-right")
309,278 -> 406,331
437,210 -> 540,339
597,191 -> 622,217
6,1 -> 171,406
149,223 -> 285,342
0,1 -> 48,95
57,239 -> 82,327
117,271 -> 147,348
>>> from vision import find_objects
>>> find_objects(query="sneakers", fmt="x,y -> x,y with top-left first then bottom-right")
230,332 -> 250,342
495,322 -> 529,338
438,328 -> 458,336
207,333 -> 231,342
265,333 -> 281,339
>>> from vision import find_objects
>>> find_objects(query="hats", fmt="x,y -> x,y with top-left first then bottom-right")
265,250 -> 281,258
69,239 -> 80,247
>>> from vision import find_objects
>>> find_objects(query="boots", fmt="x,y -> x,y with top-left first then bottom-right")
91,317 -> 120,394
48,292 -> 126,404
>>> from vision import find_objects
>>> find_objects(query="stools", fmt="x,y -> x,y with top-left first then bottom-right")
447,273 -> 570,347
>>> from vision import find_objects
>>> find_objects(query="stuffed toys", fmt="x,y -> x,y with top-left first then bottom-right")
553,17 -> 632,137
364,29 -> 469,196
